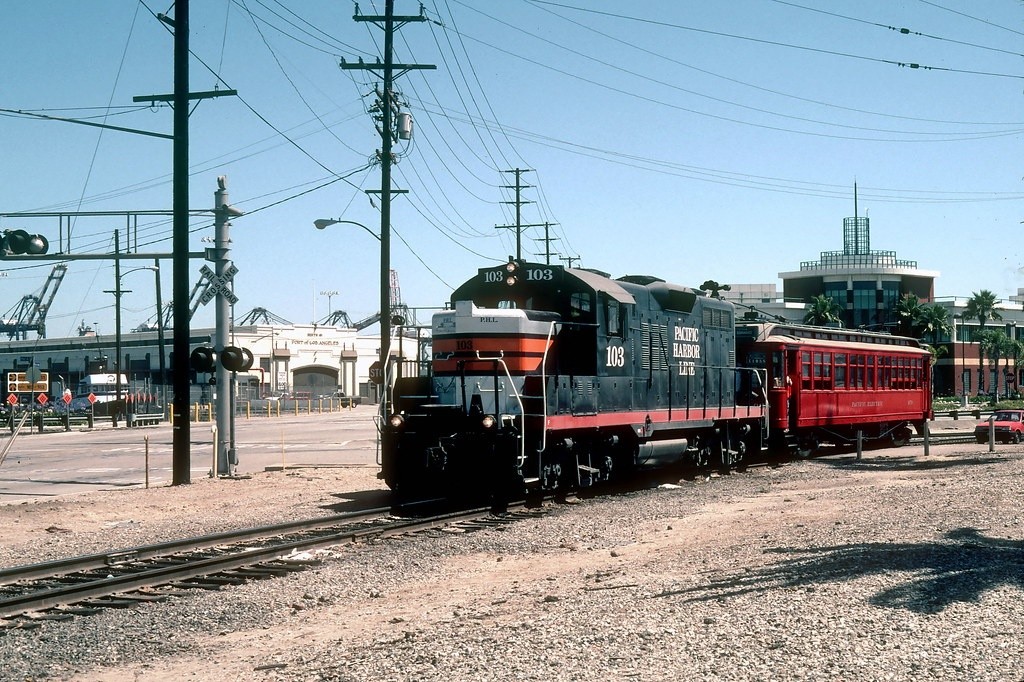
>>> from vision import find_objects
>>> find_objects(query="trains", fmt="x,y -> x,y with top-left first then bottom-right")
367,254 -> 939,499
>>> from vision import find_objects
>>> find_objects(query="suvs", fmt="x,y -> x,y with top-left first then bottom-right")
264,391 -> 288,401
281,392 -> 312,401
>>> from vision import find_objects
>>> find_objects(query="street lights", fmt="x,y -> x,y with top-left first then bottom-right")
953,313 -> 966,396
1006,321 -> 1016,399
313,219 -> 392,402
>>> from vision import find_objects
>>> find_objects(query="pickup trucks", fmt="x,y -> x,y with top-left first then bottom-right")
331,391 -> 361,408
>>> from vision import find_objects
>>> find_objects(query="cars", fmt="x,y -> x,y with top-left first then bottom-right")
973,409 -> 1024,445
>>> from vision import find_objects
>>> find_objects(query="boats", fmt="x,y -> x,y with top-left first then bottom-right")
68,374 -> 136,416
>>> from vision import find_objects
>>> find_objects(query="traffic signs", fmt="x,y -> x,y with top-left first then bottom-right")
7,372 -> 49,392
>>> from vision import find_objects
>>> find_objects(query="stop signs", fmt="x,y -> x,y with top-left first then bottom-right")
369,361 -> 386,385
1005,373 -> 1015,383
